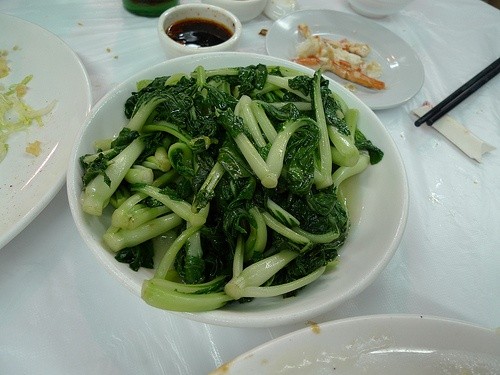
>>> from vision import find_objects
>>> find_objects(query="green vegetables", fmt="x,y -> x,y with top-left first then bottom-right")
78,63 -> 385,311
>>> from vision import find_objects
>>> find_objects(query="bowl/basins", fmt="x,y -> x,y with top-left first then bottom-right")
200,0 -> 268,23
348,0 -> 409,19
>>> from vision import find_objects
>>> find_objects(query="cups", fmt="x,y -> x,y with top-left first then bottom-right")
158,4 -> 242,58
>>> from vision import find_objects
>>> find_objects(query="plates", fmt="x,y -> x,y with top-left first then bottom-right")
266,9 -> 425,110
0,13 -> 92,250
210,314 -> 500,375
66,52 -> 408,328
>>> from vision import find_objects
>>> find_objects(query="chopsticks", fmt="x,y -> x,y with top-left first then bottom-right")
414,57 -> 500,127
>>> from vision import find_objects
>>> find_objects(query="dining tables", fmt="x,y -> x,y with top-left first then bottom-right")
0,0 -> 500,375
